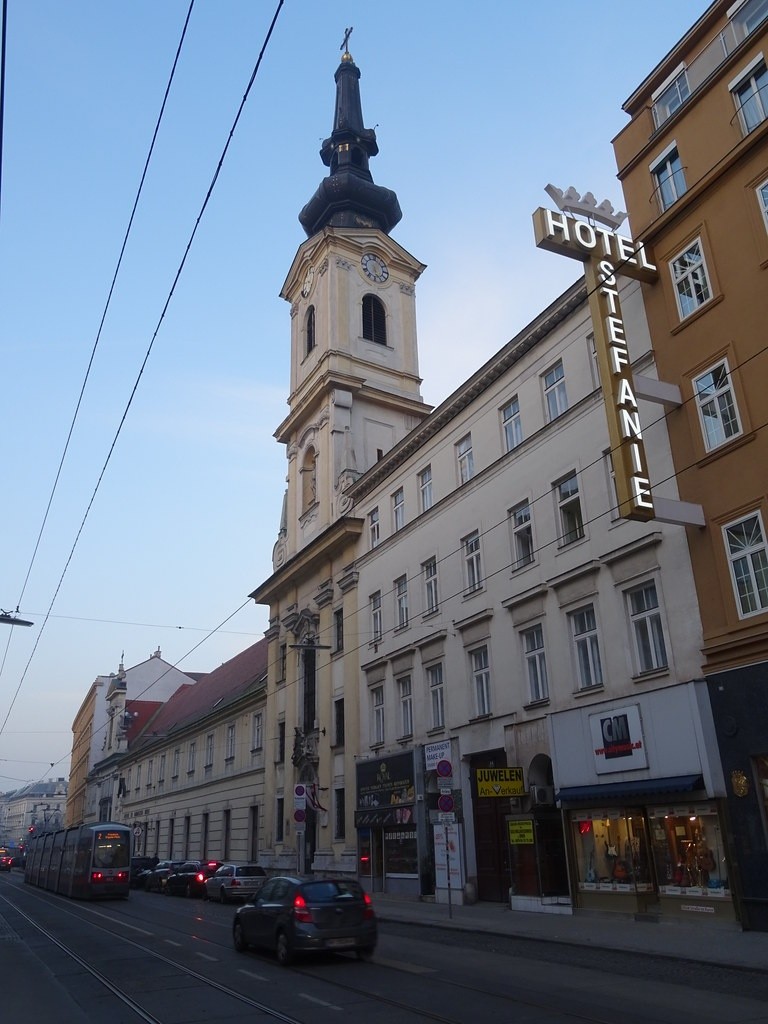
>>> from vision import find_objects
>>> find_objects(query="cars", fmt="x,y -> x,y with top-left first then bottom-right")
232,873 -> 380,967
162,858 -> 224,899
0,848 -> 15,872
202,864 -> 268,906
143,860 -> 188,893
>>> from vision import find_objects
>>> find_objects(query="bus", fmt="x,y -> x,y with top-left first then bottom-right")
22,822 -> 136,902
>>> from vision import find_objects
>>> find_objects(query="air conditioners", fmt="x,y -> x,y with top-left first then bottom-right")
530,785 -> 553,805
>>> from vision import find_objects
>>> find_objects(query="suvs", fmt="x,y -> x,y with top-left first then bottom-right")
130,855 -> 161,890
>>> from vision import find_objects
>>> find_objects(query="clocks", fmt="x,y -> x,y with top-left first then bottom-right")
361,253 -> 389,284
302,265 -> 314,297
